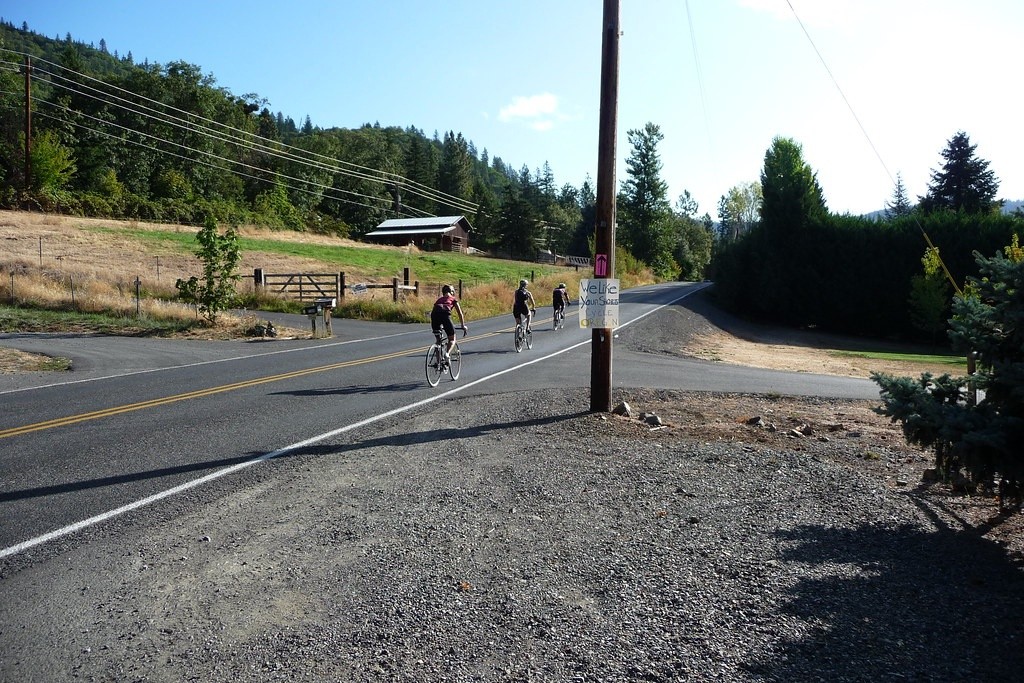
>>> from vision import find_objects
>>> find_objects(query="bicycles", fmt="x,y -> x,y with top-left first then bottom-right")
552,301 -> 571,331
425,327 -> 468,387
514,308 -> 537,353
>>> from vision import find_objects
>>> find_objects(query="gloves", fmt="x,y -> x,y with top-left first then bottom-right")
568,301 -> 571,305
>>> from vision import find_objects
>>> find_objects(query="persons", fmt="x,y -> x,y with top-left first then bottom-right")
513,280 -> 535,334
431,285 -> 468,364
553,283 -> 570,319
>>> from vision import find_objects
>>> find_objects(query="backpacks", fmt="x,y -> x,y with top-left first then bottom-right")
553,289 -> 564,299
516,289 -> 527,302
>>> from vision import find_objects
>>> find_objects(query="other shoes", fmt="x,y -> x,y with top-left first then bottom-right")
444,352 -> 451,365
526,328 -> 532,333
560,311 -> 565,317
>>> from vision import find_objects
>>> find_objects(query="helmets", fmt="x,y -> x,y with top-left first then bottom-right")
520,280 -> 528,286
559,283 -> 566,288
442,285 -> 455,293
